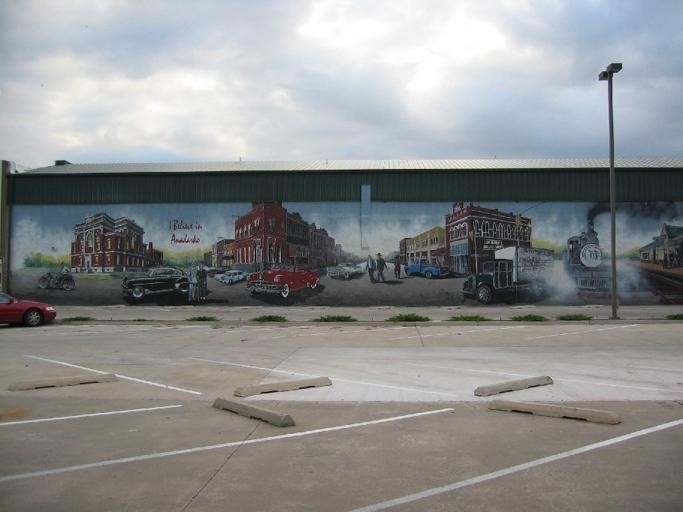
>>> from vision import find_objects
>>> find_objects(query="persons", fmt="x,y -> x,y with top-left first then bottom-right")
196,260 -> 209,301
55,262 -> 71,284
187,264 -> 197,302
394,254 -> 402,279
374,252 -> 388,283
366,252 -> 376,284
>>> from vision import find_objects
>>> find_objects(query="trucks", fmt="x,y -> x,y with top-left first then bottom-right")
461,244 -> 554,305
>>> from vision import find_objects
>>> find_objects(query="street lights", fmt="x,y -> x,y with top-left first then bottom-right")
594,61 -> 623,321
251,240 -> 257,264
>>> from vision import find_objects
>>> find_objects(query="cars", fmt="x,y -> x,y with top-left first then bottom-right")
214,270 -> 247,285
325,260 -> 361,280
243,261 -> 321,299
0,289 -> 57,329
119,266 -> 189,300
203,266 -> 226,278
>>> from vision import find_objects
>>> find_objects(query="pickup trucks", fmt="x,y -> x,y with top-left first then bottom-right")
402,258 -> 451,280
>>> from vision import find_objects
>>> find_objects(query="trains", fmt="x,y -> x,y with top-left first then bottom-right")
564,228 -> 609,293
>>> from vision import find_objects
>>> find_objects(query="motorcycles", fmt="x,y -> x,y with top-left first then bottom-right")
36,271 -> 76,291
